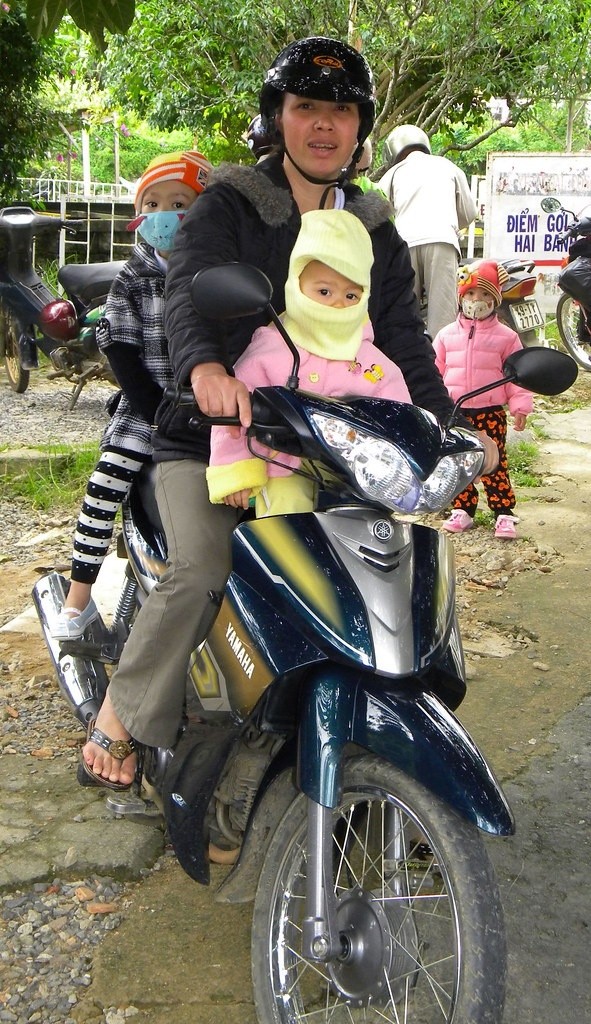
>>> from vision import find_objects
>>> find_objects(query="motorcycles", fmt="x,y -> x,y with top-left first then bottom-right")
540,195 -> 591,369
458,255 -> 544,349
30,262 -> 578,1024
0,205 -> 132,411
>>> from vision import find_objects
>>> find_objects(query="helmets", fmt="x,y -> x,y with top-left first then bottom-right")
247,36 -> 376,170
385,124 -> 431,166
40,300 -> 78,342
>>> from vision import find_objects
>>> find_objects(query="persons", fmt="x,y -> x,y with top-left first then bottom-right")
52,151 -> 215,641
376,125 -> 478,342
82,36 -> 500,790
432,259 -> 534,539
205,209 -> 413,519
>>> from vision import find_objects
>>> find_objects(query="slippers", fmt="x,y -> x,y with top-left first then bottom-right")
83,717 -> 139,791
410,841 -> 442,879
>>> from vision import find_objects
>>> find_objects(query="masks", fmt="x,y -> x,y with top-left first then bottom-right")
127,209 -> 190,251
462,298 -> 495,321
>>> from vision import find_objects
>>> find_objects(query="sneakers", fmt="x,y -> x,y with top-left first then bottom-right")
51,597 -> 98,641
444,509 -> 474,533
493,514 -> 521,540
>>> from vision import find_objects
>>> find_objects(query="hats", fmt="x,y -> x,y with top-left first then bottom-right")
281,208 -> 375,362
134,151 -> 214,216
458,259 -> 510,309
